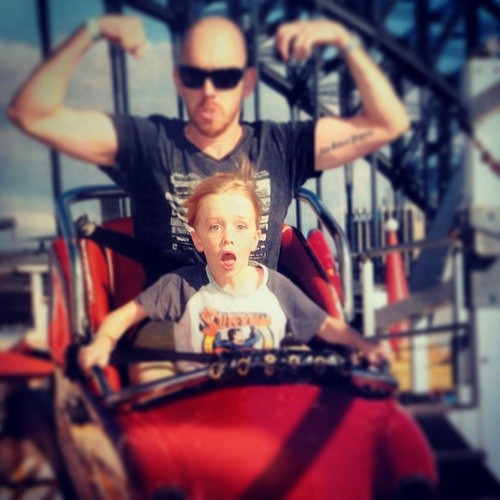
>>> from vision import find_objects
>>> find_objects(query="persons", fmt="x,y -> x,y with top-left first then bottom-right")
75,164 -> 396,371
4,16 -> 413,293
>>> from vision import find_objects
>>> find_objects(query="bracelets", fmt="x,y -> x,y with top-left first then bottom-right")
95,331 -> 117,348
82,17 -> 102,41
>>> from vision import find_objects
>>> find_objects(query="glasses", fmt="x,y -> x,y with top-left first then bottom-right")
177,64 -> 245,89
